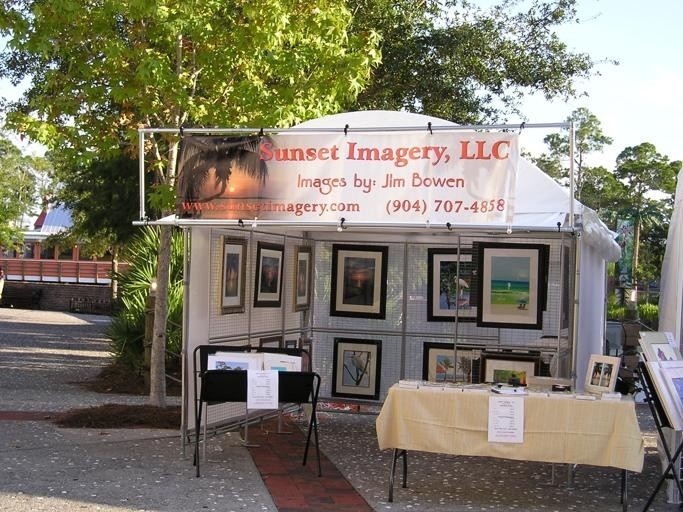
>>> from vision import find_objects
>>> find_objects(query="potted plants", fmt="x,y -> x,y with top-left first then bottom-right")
607,307 -> 626,356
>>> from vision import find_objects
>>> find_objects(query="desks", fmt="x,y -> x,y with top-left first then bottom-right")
383,381 -> 639,511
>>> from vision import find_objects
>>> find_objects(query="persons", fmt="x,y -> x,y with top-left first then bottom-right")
440,278 -> 451,310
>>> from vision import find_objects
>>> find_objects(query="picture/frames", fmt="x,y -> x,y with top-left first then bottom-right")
216,235 -> 285,317
420,341 -> 541,388
292,244 -> 312,312
329,337 -> 384,402
421,239 -> 550,329
328,242 -> 389,320
